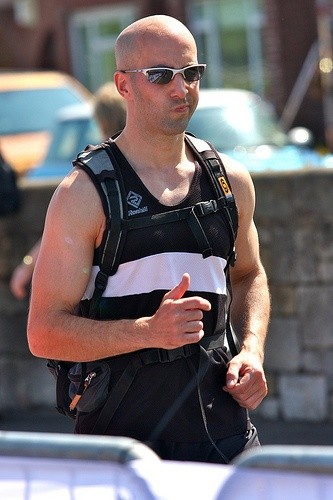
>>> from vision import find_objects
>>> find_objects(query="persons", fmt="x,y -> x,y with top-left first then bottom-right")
25,14 -> 270,467
10,82 -> 128,298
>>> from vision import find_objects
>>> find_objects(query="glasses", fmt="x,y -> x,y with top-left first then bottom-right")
115,63 -> 206,84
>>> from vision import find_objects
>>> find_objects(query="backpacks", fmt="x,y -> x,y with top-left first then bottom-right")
46,133 -> 240,419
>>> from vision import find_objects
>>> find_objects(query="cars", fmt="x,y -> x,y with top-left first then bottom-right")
0,71 -> 96,216
185,88 -> 333,173
